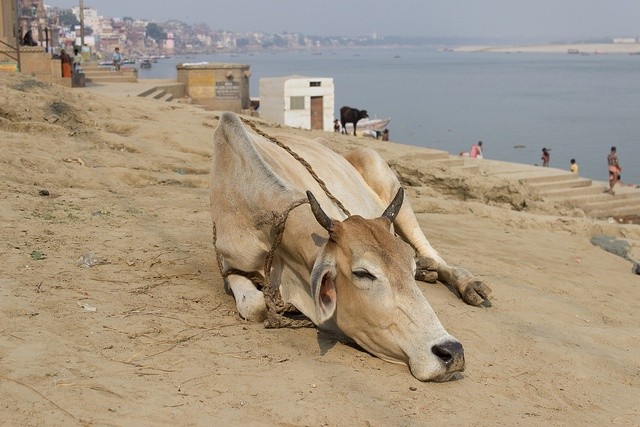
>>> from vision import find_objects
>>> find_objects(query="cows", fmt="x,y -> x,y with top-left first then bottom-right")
211,111 -> 494,383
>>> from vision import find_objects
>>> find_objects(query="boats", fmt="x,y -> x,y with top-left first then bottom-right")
338,118 -> 391,134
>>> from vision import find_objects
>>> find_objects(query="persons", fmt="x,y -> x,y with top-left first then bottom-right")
606,145 -> 622,194
334,119 -> 345,134
60,49 -> 71,78
113,47 -> 121,70
71,48 -> 83,71
540,147 -> 551,166
23,30 -> 38,47
361,130 -> 381,139
382,129 -> 390,140
476,140 -> 485,158
569,158 -> 579,173
